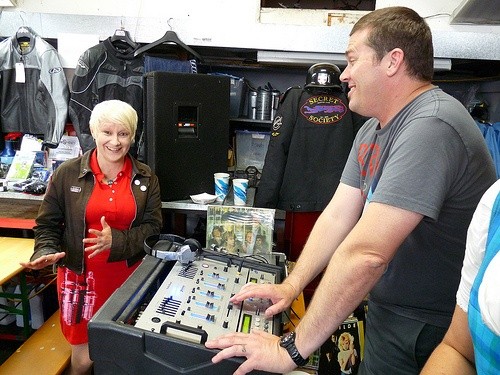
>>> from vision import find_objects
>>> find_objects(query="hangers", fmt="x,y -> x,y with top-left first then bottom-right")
17,10 -> 30,38
134,18 -> 203,61
111,16 -> 137,49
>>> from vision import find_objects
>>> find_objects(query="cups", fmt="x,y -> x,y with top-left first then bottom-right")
213,172 -> 230,202
232,178 -> 249,205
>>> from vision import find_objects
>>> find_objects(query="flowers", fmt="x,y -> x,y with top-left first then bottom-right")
5,132 -> 22,141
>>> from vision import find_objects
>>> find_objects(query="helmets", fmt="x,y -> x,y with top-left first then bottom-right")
304,63 -> 342,91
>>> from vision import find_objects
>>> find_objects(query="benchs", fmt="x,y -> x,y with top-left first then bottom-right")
0,308 -> 72,375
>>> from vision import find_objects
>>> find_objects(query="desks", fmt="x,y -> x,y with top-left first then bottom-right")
0,236 -> 35,340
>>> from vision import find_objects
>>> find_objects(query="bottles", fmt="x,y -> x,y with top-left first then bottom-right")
0,140 -> 16,166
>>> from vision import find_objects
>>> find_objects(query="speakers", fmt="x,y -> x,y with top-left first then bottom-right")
142,70 -> 230,201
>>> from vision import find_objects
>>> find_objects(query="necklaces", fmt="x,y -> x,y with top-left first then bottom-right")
105,175 -> 116,185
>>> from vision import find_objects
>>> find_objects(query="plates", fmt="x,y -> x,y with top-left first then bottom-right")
189,192 -> 222,204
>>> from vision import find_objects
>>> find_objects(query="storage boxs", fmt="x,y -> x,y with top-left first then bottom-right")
230,79 -> 248,118
234,130 -> 271,170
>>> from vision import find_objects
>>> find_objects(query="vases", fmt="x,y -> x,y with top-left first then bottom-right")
0,140 -> 15,180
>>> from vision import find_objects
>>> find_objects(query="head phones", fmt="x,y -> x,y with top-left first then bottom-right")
144,233 -> 202,264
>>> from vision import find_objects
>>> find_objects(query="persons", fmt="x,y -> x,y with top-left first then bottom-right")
419,178 -> 500,375
205,7 -> 497,375
324,332 -> 358,375
19,100 -> 163,375
208,226 -> 270,259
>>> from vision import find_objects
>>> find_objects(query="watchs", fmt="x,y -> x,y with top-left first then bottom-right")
279,331 -> 309,367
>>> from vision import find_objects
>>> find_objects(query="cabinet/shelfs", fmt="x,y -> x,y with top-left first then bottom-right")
0,116 -> 287,220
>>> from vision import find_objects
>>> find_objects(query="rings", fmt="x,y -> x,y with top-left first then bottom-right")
241,344 -> 246,356
